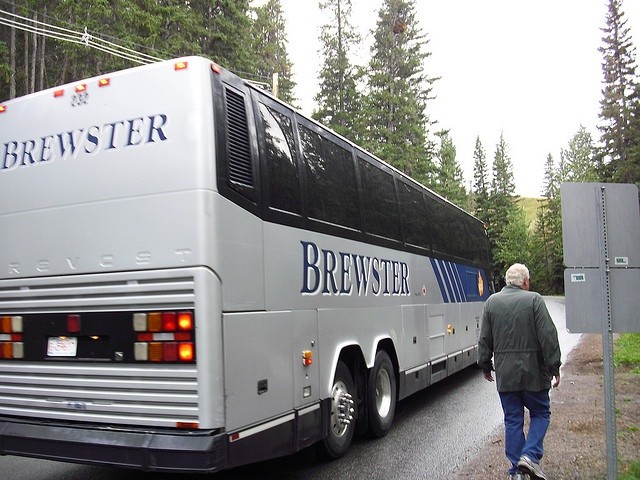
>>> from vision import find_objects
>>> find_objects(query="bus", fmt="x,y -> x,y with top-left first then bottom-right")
0,56 -> 505,473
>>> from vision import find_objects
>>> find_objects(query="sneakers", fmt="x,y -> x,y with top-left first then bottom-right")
509,473 -> 526,480
517,455 -> 547,479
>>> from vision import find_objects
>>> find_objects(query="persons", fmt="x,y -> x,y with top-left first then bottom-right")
478,263 -> 562,480
